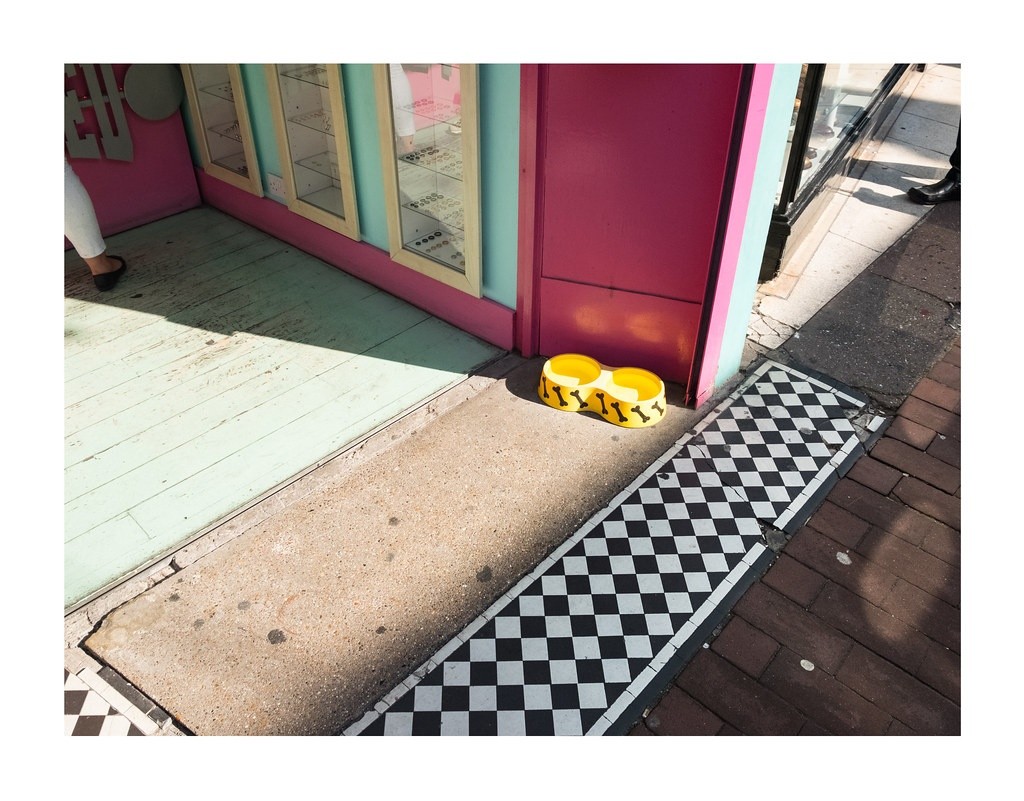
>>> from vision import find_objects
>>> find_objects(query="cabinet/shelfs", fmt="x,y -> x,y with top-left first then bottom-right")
198,82 -> 241,144
281,67 -> 341,184
396,97 -> 466,231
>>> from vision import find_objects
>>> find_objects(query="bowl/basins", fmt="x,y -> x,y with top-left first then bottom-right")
536,352 -> 667,429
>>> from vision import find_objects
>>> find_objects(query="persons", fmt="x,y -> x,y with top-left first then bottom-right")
65,158 -> 126,292
390,64 -> 422,164
908,118 -> 961,204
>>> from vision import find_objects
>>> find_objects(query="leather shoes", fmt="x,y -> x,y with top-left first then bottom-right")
907,168 -> 961,205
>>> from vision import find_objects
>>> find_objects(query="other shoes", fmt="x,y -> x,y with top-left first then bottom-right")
92,256 -> 127,291
449,121 -> 461,135
